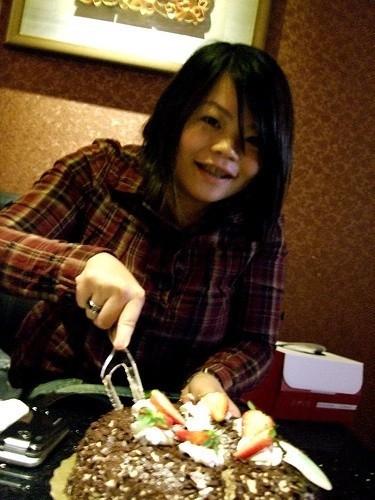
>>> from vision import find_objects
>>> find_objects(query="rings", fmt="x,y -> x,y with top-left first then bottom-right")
88,300 -> 102,313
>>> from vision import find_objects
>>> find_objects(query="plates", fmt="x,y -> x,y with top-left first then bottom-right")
48,436 -> 335,500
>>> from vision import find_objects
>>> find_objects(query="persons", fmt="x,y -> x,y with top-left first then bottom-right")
0,41 -> 296,422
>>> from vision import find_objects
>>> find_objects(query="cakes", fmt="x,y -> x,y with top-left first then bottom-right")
64,391 -> 333,499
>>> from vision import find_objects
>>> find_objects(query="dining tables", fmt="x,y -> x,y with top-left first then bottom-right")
0,394 -> 375,499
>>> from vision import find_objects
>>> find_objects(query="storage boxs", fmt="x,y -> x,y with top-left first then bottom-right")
239,346 -> 363,423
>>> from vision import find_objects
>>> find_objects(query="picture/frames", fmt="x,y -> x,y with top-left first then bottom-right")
5,1 -> 274,82
